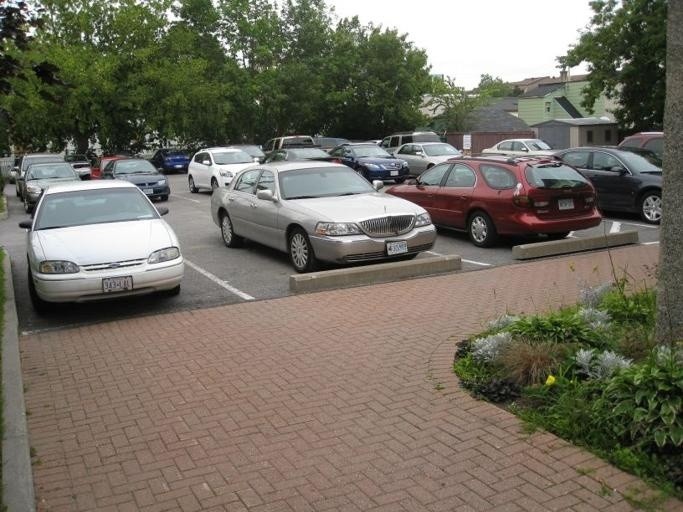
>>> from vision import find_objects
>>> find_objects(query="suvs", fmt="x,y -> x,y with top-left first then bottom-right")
618,131 -> 662,159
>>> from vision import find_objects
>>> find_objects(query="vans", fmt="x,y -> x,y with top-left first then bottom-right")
377,130 -> 441,154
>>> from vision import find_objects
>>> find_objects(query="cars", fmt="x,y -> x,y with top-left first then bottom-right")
20,180 -> 183,309
212,161 -> 436,272
187,149 -> 260,191
551,146 -> 661,225
230,136 -> 466,182
385,156 -> 602,247
150,150 -> 190,172
480,138 -> 561,155
9,153 -> 171,202
